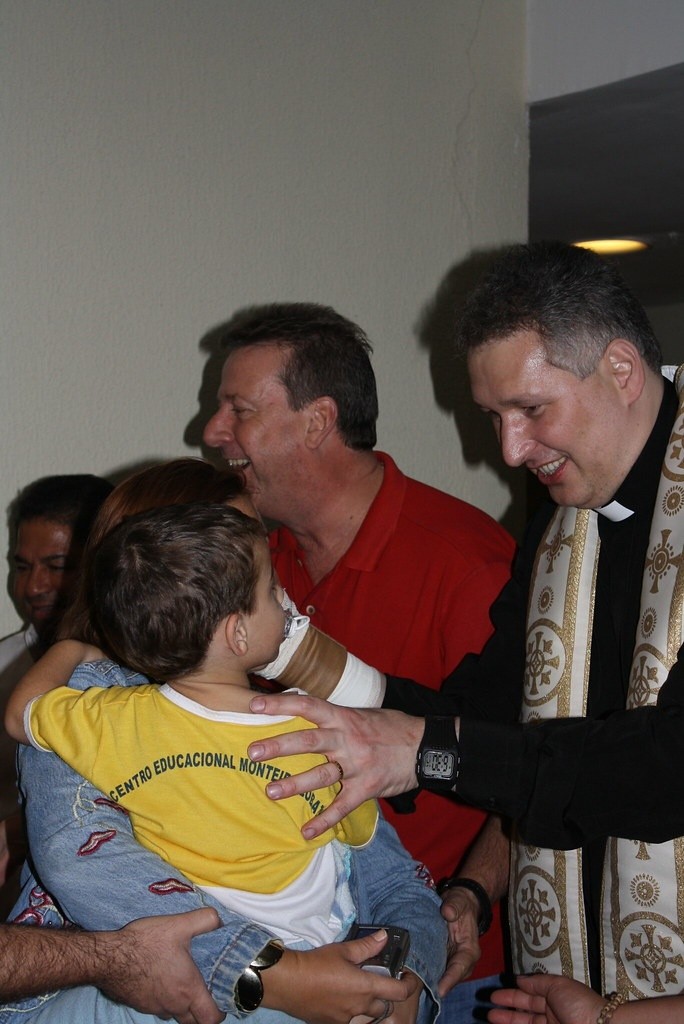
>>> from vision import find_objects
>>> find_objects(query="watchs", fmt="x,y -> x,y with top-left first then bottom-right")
415,715 -> 460,793
438,879 -> 493,936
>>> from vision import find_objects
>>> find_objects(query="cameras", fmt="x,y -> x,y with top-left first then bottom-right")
344,923 -> 410,981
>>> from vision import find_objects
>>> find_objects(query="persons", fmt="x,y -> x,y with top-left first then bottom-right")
0,245 -> 684,1024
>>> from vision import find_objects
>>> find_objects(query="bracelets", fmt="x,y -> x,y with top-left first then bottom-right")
232,939 -> 284,1014
597,991 -> 625,1024
333,761 -> 343,779
